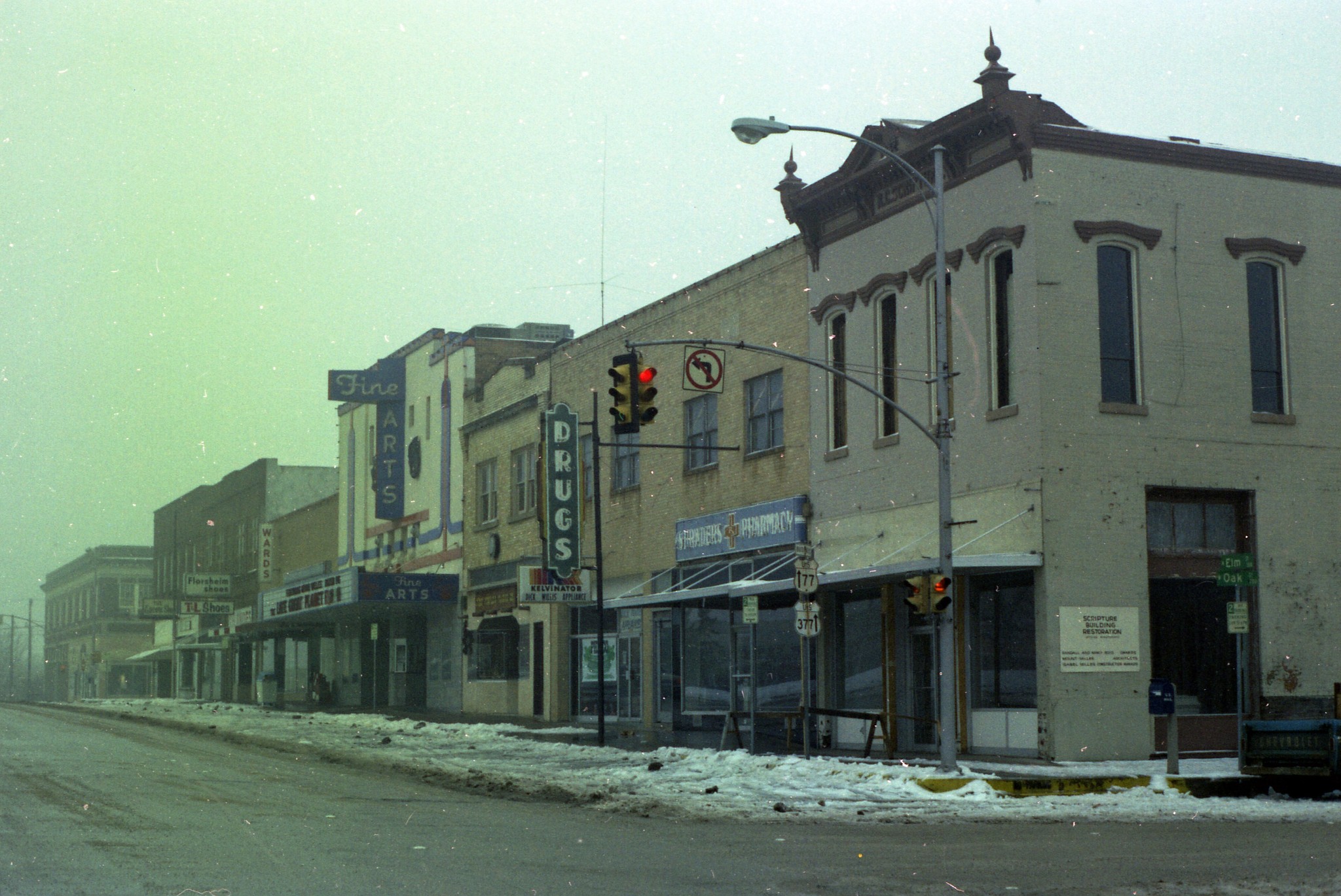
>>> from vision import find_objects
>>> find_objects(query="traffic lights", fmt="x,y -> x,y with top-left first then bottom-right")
605,351 -> 659,433
907,572 -> 950,617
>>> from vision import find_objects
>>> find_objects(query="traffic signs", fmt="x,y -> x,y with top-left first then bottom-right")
1215,554 -> 1258,590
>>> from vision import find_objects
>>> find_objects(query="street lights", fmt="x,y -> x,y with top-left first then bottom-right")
730,113 -> 963,773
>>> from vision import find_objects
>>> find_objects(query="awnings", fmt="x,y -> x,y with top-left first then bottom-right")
597,550 -> 1044,611
124,647 -> 173,663
